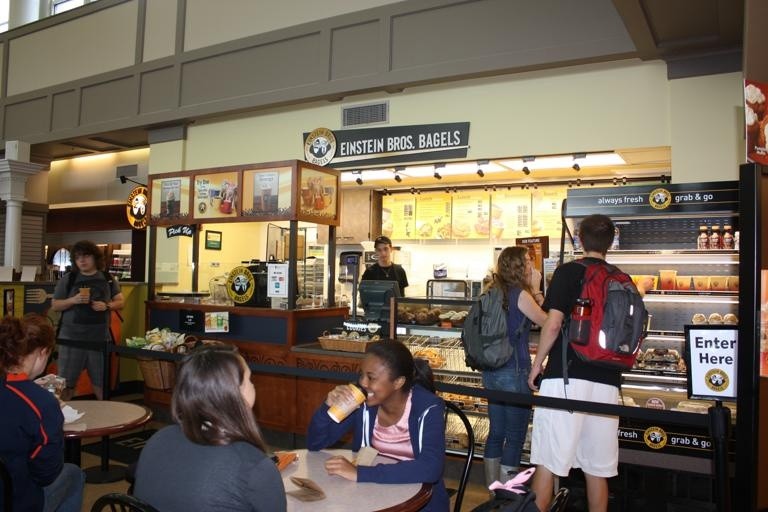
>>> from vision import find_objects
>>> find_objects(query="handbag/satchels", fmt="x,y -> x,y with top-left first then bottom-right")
470,486 -> 541,512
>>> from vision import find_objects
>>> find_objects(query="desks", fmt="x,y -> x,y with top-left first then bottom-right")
275,448 -> 433,512
62,399 -> 152,484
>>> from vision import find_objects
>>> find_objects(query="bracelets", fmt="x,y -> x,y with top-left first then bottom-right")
531,290 -> 544,296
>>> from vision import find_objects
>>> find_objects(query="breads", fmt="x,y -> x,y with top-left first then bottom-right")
400,307 -> 468,326
691,312 -> 739,326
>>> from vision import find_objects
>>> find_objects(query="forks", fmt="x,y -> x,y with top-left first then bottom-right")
24,287 -> 53,305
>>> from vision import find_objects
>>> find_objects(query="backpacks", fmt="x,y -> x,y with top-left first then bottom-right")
461,273 -> 527,370
561,259 -> 648,371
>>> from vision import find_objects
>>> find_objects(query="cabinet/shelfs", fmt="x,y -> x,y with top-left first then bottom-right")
558,180 -> 742,512
285,257 -> 324,296
317,190 -> 382,244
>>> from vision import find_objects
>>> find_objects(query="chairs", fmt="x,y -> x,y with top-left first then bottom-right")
443,399 -> 474,512
91,492 -> 159,512
0,454 -> 15,512
546,487 -> 570,512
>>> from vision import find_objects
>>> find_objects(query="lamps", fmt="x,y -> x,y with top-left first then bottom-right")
351,153 -> 671,195
119,175 -> 147,187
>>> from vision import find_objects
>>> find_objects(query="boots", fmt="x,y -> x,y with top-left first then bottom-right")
482,456 -> 520,496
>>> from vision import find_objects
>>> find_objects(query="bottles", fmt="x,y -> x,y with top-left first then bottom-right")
608,221 -> 620,251
572,297 -> 592,316
573,221 -> 582,251
696,224 -> 739,249
205,314 -> 228,330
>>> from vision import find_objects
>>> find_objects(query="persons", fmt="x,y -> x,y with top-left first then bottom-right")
480,245 -> 549,498
527,215 -> 626,512
131,343 -> 287,512
1,314 -> 87,511
307,337 -> 451,511
360,239 -> 408,297
50,239 -> 127,404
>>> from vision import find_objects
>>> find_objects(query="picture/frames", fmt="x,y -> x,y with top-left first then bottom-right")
205,230 -> 222,250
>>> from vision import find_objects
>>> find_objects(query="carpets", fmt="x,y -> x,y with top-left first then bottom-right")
81,429 -> 158,464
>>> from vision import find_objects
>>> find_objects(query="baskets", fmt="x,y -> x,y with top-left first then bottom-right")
136,335 -> 224,390
317,331 -> 380,354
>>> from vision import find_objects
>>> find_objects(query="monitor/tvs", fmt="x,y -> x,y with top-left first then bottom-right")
358,280 -> 401,307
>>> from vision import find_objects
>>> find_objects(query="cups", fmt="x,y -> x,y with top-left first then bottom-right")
79,287 -> 90,304
260,188 -> 272,212
302,186 -> 333,209
209,196 -> 232,212
167,199 -> 175,215
327,383 -> 368,424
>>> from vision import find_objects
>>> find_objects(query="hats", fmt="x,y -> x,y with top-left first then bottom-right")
374,237 -> 391,248
